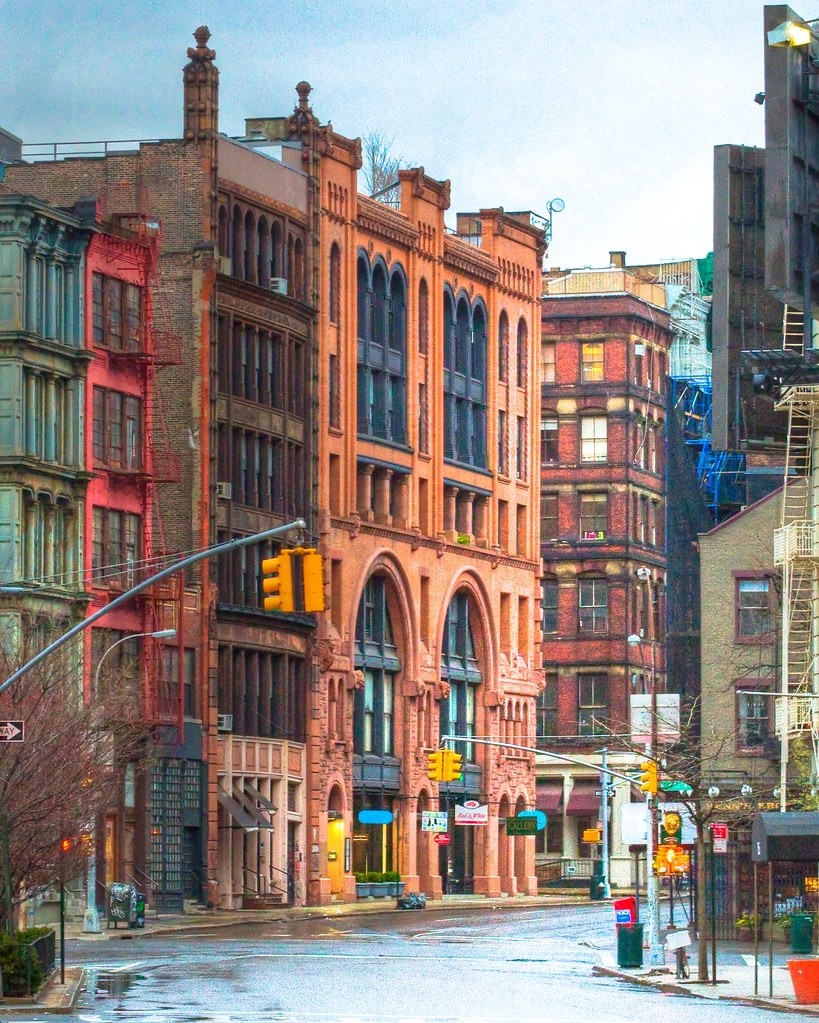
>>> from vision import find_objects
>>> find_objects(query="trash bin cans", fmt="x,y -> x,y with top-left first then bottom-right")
787,912 -> 817,953
615,922 -> 646,967
129,893 -> 146,928
589,875 -> 607,901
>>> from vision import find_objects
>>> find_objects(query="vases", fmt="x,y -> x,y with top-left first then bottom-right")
736,925 -> 759,943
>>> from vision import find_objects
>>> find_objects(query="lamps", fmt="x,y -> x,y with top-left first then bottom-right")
767,17 -> 819,48
774,780 -> 781,798
741,779 -> 753,795
754,92 -> 765,105
707,779 -> 720,797
270,277 -> 288,295
217,482 -> 232,501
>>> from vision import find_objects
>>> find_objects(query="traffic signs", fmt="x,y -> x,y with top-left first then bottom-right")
594,787 -> 616,799
0,719 -> 26,743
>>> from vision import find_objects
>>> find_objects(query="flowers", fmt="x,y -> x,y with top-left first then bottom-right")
734,908 -> 764,928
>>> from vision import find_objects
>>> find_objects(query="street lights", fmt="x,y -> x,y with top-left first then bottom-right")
80,627 -> 179,935
627,565 -> 661,875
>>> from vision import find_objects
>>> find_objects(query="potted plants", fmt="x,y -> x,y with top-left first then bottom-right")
384,871 -> 406,897
366,872 -> 389,897
775,912 -> 792,944
353,872 -> 370,897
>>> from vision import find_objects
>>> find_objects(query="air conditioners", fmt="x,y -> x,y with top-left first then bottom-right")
218,714 -> 233,731
635,345 -> 646,357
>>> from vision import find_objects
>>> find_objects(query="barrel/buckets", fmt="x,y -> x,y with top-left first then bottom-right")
786,959 -> 819,1003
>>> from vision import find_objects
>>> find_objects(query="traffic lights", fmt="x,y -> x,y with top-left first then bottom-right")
640,760 -> 658,795
303,554 -> 330,612
426,749 -> 444,783
442,749 -> 465,782
60,838 -> 72,855
260,554 -> 294,614
